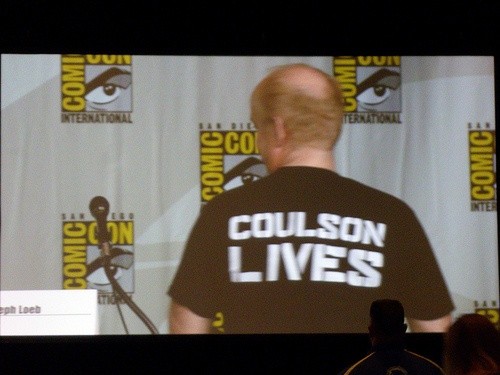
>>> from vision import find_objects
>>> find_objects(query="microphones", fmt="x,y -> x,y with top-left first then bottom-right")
90,195 -> 111,261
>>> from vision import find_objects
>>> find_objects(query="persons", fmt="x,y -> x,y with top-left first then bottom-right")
344,299 -> 446,375
443,313 -> 500,375
166,63 -> 456,333
